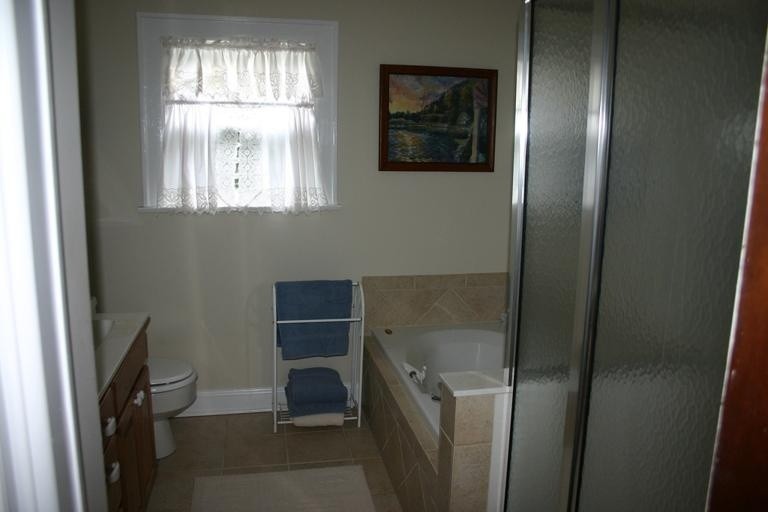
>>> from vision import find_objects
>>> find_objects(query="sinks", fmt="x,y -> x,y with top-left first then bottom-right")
92,317 -> 115,349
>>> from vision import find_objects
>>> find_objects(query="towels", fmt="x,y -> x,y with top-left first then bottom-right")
275,281 -> 353,428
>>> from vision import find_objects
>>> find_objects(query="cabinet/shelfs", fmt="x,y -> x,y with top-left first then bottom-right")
98,330 -> 158,511
271,278 -> 366,432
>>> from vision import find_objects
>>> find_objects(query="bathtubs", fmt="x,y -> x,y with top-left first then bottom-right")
370,320 -> 507,448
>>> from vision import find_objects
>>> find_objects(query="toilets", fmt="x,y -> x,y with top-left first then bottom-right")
148,358 -> 200,460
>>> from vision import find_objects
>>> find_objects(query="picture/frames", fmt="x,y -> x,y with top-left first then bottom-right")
377,63 -> 498,174
